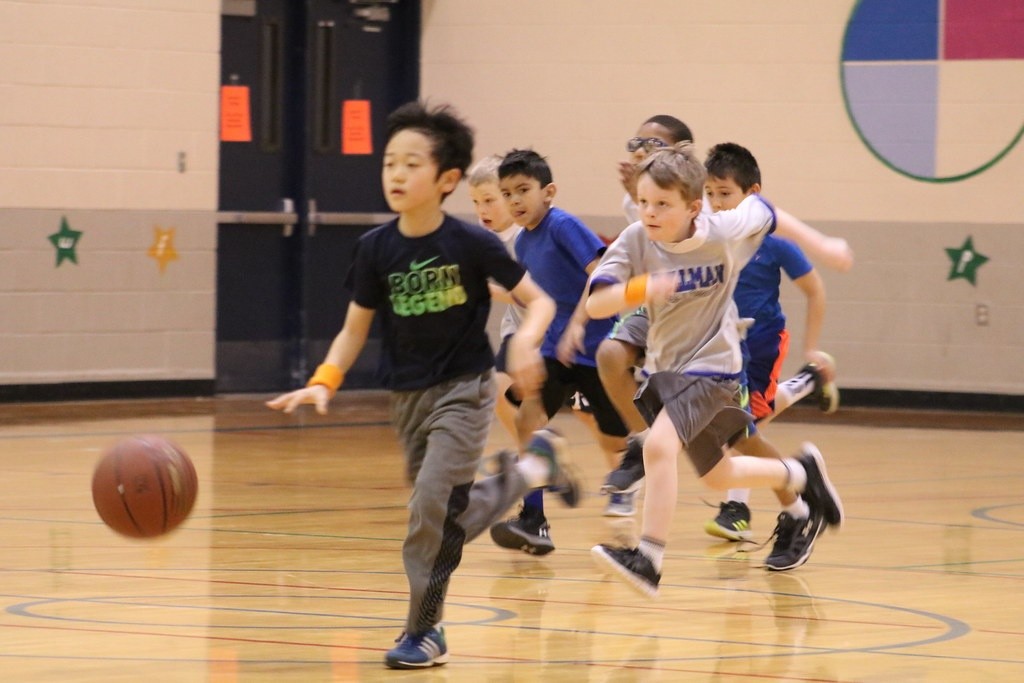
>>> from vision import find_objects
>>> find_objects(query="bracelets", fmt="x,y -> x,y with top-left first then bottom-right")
306,363 -> 344,399
625,273 -> 647,307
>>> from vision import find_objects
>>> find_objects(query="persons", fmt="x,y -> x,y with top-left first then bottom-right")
467,114 -> 855,595
264,97 -> 583,670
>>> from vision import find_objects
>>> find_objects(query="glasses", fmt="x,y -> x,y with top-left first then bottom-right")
626,136 -> 671,156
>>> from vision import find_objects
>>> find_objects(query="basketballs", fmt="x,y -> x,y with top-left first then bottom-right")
89,434 -> 199,540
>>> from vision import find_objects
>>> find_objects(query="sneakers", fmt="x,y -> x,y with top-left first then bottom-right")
702,501 -> 759,544
600,434 -> 646,495
488,515 -> 556,557
523,428 -> 584,508
794,440 -> 843,529
801,350 -> 839,414
590,543 -> 664,597
600,490 -> 639,516
383,625 -> 450,669
762,498 -> 827,571
